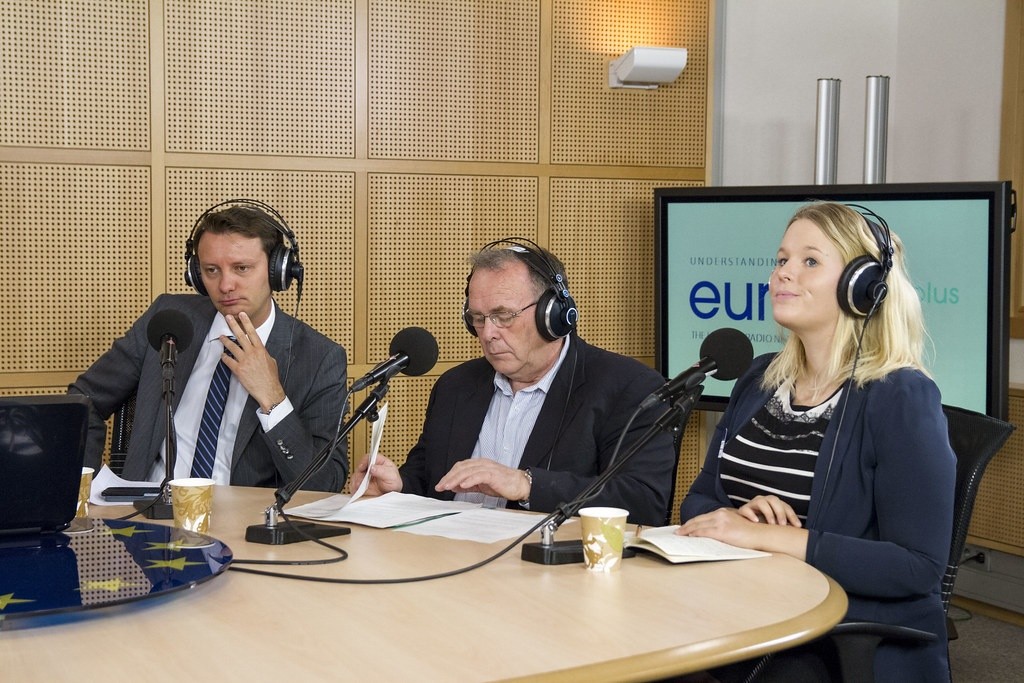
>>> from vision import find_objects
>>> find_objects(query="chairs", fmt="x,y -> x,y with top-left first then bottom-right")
110,390 -> 137,484
666,378 -> 704,526
748,403 -> 1017,682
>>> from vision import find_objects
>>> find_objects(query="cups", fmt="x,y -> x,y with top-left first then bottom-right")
168,477 -> 215,535
75,467 -> 95,518
578,506 -> 630,574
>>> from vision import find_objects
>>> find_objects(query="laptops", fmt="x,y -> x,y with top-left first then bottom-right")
0,394 -> 90,537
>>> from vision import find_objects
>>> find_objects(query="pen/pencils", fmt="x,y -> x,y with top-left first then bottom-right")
634,524 -> 643,538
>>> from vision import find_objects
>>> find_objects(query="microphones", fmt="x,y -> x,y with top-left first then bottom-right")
350,326 -> 439,393
637,328 -> 754,412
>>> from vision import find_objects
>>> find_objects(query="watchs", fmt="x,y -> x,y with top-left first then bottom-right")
266,403 -> 279,416
523,469 -> 533,486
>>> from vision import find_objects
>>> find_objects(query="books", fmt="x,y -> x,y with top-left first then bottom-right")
621,519 -> 773,563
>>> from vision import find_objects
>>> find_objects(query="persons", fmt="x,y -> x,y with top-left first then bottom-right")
347,235 -> 677,529
67,194 -> 350,494
675,201 -> 959,683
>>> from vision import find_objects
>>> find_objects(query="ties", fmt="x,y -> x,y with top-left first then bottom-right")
190,337 -> 244,478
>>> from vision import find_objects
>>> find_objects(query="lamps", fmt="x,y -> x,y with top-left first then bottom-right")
610,42 -> 690,93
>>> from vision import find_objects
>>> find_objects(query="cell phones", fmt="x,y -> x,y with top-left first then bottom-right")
101,486 -> 159,502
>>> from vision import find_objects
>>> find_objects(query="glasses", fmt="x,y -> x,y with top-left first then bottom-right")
463,300 -> 537,328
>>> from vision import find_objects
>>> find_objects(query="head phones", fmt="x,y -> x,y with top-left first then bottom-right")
837,203 -> 894,318
462,237 -> 578,341
184,198 -> 305,296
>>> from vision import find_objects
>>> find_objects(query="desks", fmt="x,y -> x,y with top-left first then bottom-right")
0,480 -> 847,682
